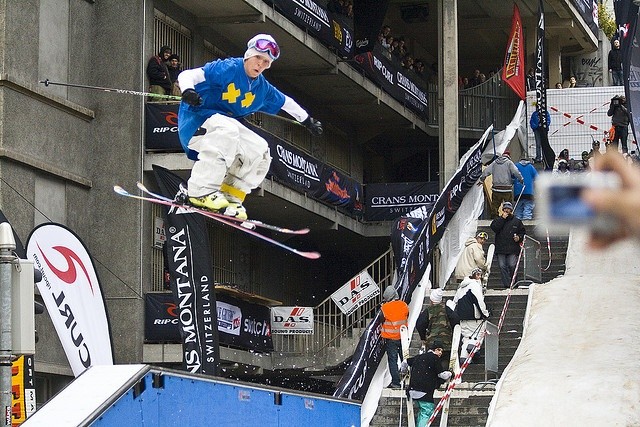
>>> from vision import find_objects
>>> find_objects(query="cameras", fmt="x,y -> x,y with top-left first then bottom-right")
536,169 -> 624,234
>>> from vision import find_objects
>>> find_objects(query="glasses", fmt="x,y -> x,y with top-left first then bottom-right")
248,39 -> 280,60
477,232 -> 488,239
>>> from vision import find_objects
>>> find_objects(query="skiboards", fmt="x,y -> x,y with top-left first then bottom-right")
138,178 -> 322,261
114,181 -> 311,236
481,244 -> 495,288
512,185 -> 525,214
437,324 -> 461,427
398,325 -> 416,427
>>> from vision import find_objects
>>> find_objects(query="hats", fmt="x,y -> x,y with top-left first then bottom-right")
431,341 -> 444,352
430,288 -> 442,302
502,202 -> 514,211
471,269 -> 481,276
244,34 -> 280,69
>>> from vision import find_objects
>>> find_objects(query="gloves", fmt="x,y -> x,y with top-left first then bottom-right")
182,88 -> 201,106
299,116 -> 323,137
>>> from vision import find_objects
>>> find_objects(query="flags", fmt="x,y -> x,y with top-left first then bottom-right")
500,1 -> 525,102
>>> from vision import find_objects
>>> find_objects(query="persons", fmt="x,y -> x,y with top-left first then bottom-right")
555,82 -> 563,89
608,40 -> 623,86
460,70 -> 495,89
554,159 -> 571,177
558,149 -> 569,161
573,152 -> 590,171
178,34 -> 322,220
607,95 -> 631,152
377,285 -> 409,388
402,343 -> 446,427
582,152 -> 640,250
378,27 -> 425,74
588,140 -> 602,172
477,151 -> 524,220
453,268 -> 490,367
168,54 -> 181,84
416,288 -> 463,371
147,45 -> 174,101
530,104 -> 554,161
490,202 -> 526,289
454,231 -> 489,283
568,76 -> 578,86
513,157 -> 540,220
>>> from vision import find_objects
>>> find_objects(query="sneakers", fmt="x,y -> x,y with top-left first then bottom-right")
229,202 -> 247,220
189,192 -> 229,209
